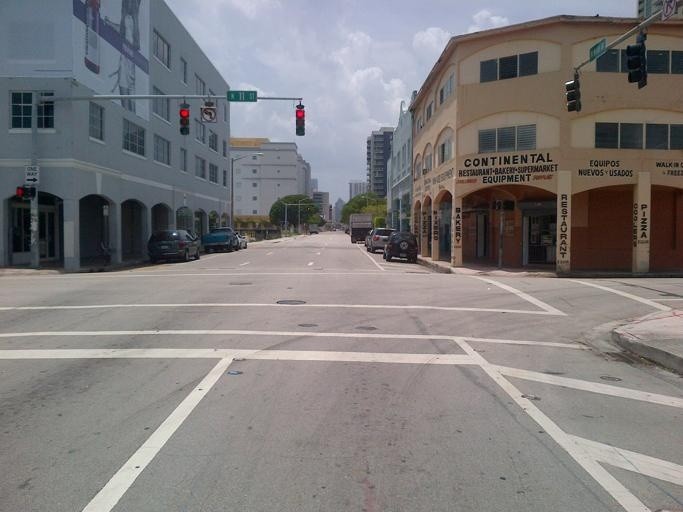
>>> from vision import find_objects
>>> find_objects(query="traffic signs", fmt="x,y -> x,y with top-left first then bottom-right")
637,0 -> 664,23
24,164 -> 40,185
226,91 -> 257,103
589,36 -> 607,65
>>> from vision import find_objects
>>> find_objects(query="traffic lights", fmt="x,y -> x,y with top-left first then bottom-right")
15,186 -> 37,199
179,102 -> 190,135
564,71 -> 581,113
624,32 -> 648,90
295,109 -> 305,136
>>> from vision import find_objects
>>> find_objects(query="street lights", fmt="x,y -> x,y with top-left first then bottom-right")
229,153 -> 264,227
360,196 -> 378,207
297,196 -> 310,234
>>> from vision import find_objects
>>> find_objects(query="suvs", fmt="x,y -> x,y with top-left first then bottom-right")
364,227 -> 418,264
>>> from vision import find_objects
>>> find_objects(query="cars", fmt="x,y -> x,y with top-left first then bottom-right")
308,228 -> 319,235
148,229 -> 201,262
236,234 -> 247,250
202,227 -> 238,253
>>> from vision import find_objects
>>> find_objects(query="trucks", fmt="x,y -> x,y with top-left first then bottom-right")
349,213 -> 372,243
